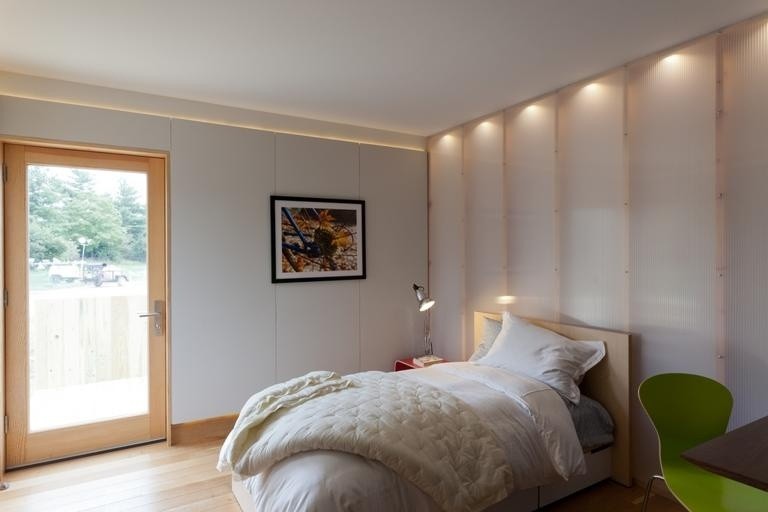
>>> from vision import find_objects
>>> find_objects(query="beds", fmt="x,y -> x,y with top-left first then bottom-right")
227,311 -> 640,512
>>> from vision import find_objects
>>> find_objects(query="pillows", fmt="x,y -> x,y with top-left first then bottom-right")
467,311 -> 607,406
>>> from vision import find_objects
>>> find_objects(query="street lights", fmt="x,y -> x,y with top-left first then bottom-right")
76,236 -> 93,284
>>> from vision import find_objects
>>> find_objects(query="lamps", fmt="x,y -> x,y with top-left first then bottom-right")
411,284 -> 436,317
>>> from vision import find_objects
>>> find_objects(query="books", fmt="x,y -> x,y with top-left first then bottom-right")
412,354 -> 443,368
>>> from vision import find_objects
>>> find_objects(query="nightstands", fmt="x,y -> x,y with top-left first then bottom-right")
394,354 -> 442,372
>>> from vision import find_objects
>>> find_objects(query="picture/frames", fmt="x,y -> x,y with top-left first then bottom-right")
268,193 -> 367,285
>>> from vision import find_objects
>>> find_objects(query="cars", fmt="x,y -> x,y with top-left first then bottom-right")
47,260 -> 92,284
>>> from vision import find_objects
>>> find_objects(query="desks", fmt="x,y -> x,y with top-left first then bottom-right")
683,414 -> 767,495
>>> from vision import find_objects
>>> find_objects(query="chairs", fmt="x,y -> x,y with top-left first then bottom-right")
638,372 -> 768,512
